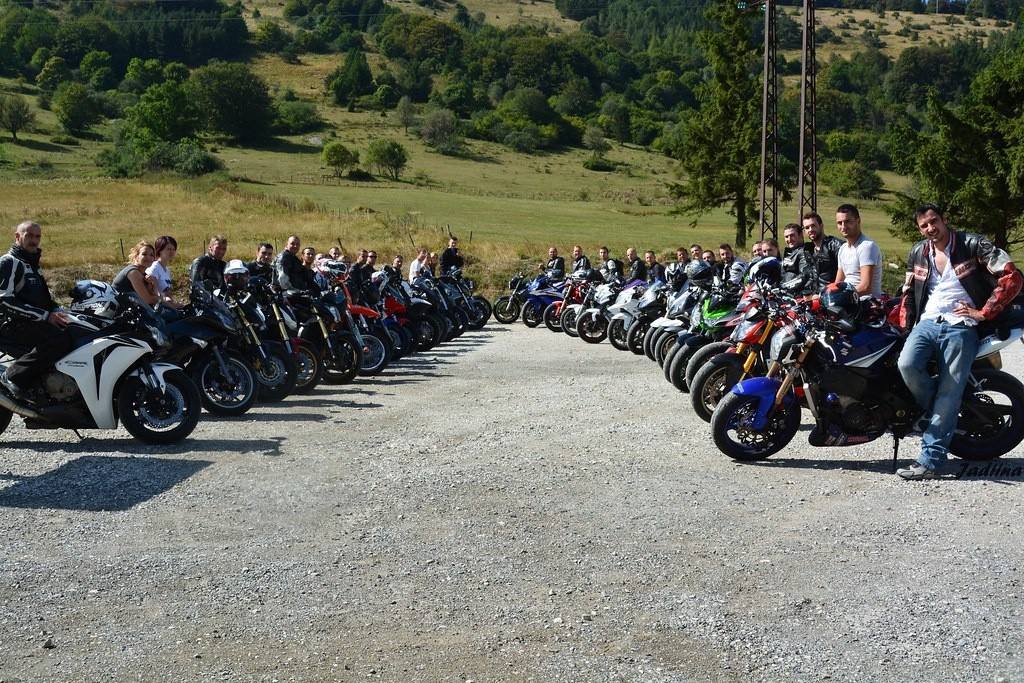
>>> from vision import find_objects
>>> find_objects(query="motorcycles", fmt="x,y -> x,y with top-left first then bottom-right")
221,265 -> 492,404
0,291 -> 202,446
157,274 -> 260,417
709,299 -> 1024,461
493,267 -> 825,425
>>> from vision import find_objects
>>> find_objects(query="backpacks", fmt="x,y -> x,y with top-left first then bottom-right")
605,259 -> 623,276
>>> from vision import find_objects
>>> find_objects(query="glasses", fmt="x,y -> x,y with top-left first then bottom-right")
370,255 -> 377,259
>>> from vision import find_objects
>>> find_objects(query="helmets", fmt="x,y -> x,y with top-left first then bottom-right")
664,262 -> 683,283
224,259 -> 250,290
750,256 -> 783,288
371,271 -> 387,283
69,279 -> 119,320
686,259 -> 712,278
819,281 -> 859,320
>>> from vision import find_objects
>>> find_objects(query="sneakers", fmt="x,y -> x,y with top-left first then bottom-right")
896,461 -> 942,480
0,380 -> 38,418
912,411 -> 933,432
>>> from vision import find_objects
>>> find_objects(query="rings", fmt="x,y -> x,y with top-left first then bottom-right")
963,305 -> 966,309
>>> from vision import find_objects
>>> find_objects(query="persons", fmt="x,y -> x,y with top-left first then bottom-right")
274,235 -> 306,294
440,236 -> 463,277
385,255 -> 403,287
897,204 -> 1023,478
645,251 -> 665,278
600,246 -> 617,281
301,247 -> 315,295
409,248 -> 439,286
667,243 -> 749,287
752,241 -> 762,257
538,247 -> 564,280
0,220 -> 68,405
802,213 -> 845,292
189,235 -> 227,309
626,247 -> 647,284
145,236 -> 177,304
110,240 -> 162,308
780,223 -> 819,290
572,245 -> 590,273
825,204 -> 882,302
246,243 -> 278,285
762,238 -> 782,261
315,246 -> 377,299
